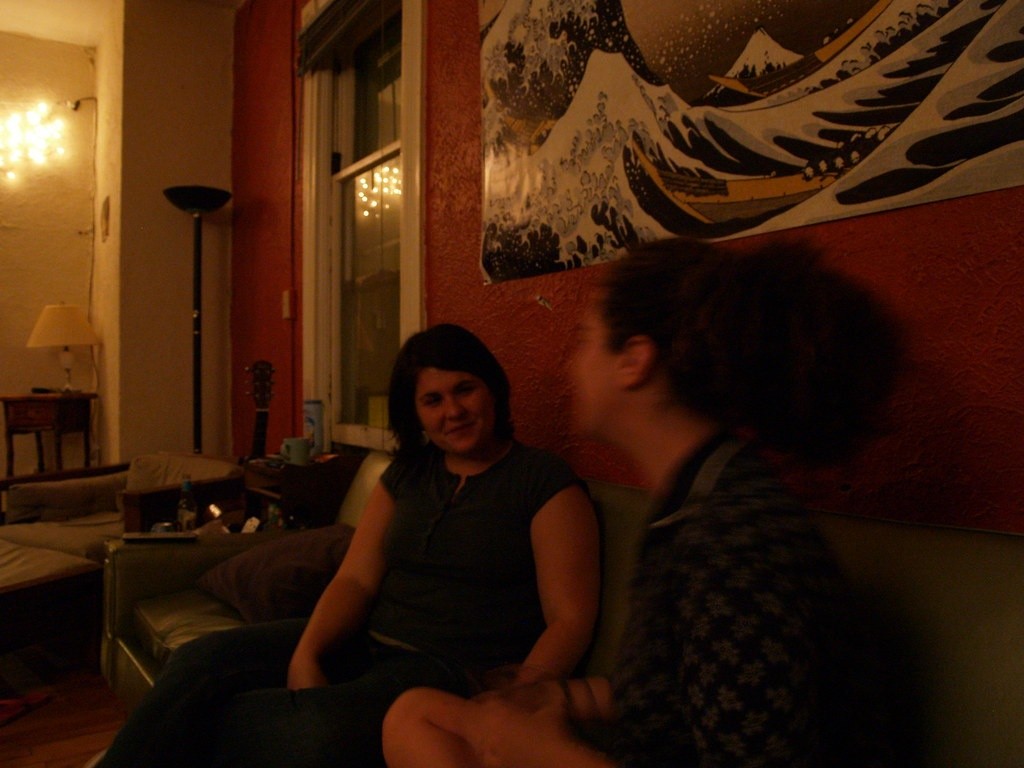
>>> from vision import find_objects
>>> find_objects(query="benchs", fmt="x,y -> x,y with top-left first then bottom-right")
0,452 -> 243,655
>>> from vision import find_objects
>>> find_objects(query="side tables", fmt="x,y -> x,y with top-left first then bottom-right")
244,455 -> 362,531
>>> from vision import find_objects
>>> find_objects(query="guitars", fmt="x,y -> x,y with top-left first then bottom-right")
239,359 -> 277,467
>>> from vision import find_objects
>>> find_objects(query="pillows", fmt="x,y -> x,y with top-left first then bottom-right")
197,523 -> 356,624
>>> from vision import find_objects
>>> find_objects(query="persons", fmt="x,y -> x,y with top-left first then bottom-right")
381,238 -> 898,768
92,323 -> 602,768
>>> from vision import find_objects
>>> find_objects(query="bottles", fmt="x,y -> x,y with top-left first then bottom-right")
175,473 -> 198,533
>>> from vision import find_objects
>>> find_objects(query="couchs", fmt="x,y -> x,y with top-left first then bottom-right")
100,451 -> 1024,768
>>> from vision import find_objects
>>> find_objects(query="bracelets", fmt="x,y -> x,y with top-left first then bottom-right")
581,675 -> 600,720
557,674 -> 579,722
519,662 -> 552,678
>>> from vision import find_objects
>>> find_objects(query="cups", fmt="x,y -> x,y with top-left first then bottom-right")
484,662 -> 562,715
281,436 -> 310,467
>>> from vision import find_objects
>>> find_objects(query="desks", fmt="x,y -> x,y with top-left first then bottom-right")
0,392 -> 97,478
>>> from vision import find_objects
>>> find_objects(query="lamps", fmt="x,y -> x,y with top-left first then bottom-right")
26,301 -> 101,392
164,185 -> 234,455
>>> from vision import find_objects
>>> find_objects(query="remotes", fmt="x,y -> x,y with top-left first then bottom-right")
122,533 -> 197,544
